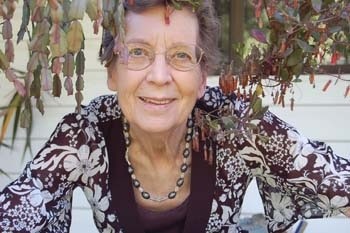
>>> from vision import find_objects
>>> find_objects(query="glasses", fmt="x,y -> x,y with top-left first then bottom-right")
114,43 -> 205,71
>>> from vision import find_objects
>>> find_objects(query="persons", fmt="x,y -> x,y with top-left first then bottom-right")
0,0 -> 350,233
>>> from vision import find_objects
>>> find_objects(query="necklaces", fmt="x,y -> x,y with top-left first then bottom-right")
123,111 -> 193,202
